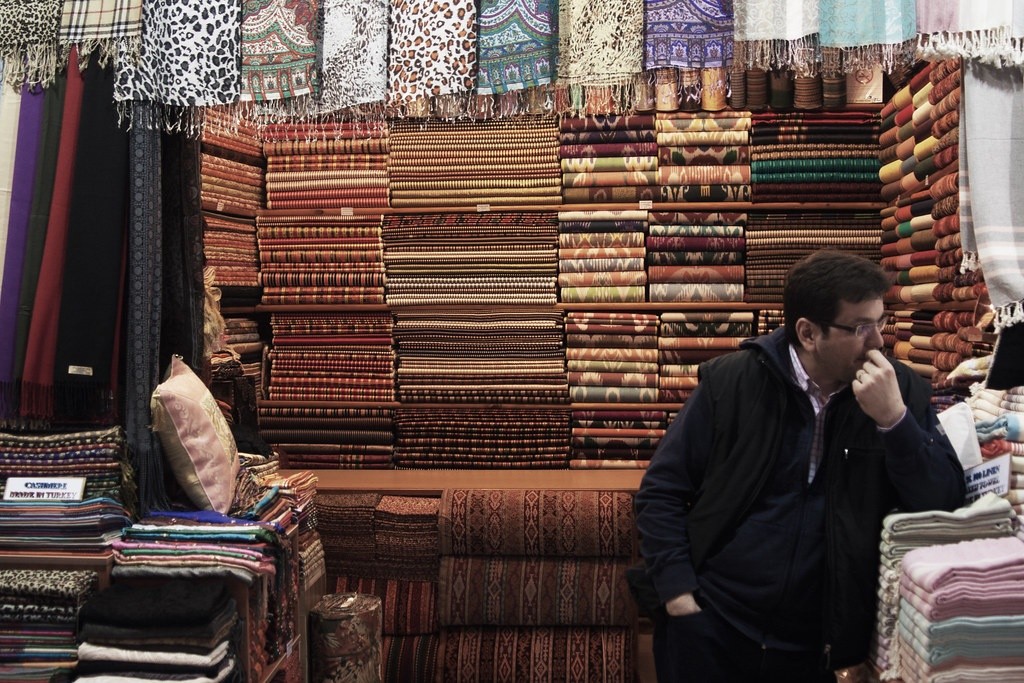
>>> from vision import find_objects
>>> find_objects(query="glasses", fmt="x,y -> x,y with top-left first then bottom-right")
808,313 -> 889,340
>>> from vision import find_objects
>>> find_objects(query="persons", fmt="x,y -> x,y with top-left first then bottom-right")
624,250 -> 967,683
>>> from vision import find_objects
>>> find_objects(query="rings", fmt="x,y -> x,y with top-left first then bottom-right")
858,372 -> 867,383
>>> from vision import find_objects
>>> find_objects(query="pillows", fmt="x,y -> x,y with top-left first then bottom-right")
152,352 -> 239,515
